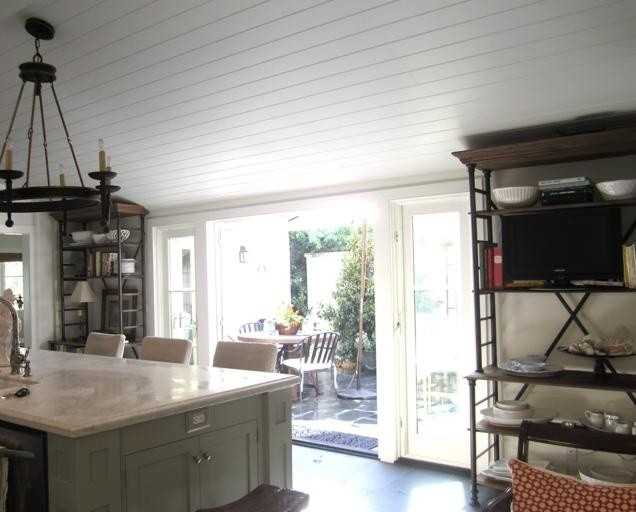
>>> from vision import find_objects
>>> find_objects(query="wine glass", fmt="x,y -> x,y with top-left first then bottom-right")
559,342 -> 636,386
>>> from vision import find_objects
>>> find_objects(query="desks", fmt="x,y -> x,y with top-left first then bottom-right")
235,329 -> 339,399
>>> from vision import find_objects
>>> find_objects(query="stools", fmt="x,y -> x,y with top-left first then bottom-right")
195,481 -> 312,511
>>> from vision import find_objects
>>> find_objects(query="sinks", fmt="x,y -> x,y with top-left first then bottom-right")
0,372 -> 41,392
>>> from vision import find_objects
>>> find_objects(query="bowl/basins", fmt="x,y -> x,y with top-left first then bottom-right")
594,178 -> 636,203
480,396 -> 558,427
493,186 -> 538,208
577,463 -> 634,487
70,230 -> 129,244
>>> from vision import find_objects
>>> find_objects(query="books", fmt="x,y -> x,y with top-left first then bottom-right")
87,250 -> 125,278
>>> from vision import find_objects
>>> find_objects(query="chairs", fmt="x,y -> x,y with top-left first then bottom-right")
239,321 -> 262,333
211,340 -> 281,374
484,414 -> 635,512
283,331 -> 342,404
137,334 -> 196,363
81,331 -> 126,358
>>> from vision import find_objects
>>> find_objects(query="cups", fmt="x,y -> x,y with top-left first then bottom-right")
498,354 -> 564,378
582,406 -> 636,436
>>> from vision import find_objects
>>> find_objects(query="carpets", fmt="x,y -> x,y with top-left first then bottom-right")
293,430 -> 377,450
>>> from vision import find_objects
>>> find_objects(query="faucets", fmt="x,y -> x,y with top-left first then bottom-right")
1,297 -> 22,374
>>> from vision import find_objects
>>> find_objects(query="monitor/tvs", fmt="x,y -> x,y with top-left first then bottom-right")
502,201 -> 623,290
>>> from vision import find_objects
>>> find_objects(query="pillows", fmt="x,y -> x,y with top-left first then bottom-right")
506,456 -> 636,511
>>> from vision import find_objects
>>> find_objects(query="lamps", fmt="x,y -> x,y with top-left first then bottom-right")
238,244 -> 248,264
69,278 -> 93,341
1,16 -> 116,234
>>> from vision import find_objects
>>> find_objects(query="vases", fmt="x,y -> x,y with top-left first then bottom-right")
278,323 -> 297,335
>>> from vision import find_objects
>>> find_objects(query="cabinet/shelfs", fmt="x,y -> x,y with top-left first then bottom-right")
126,415 -> 257,510
57,207 -> 153,345
449,116 -> 636,512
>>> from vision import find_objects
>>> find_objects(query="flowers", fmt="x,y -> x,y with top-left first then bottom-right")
274,304 -> 304,327
